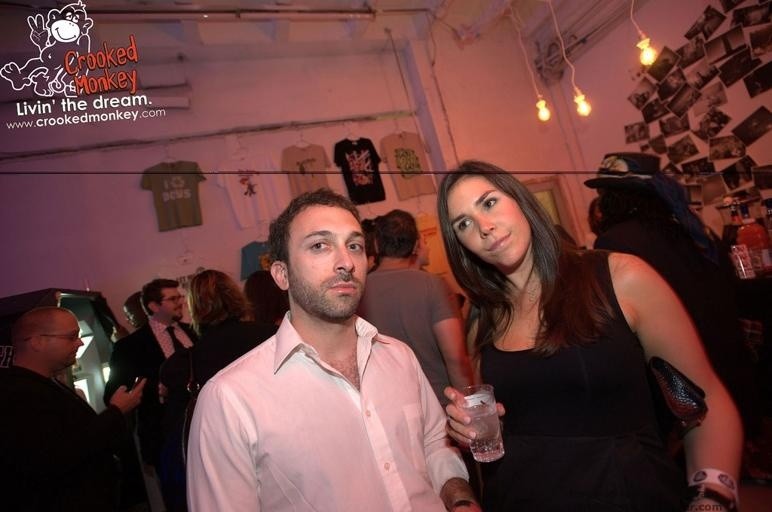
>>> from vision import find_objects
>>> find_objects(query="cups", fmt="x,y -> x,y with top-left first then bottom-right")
451,384 -> 506,464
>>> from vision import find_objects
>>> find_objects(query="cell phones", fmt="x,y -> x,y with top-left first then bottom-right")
127,376 -> 139,390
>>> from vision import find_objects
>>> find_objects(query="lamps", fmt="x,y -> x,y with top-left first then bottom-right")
504,1 -> 659,123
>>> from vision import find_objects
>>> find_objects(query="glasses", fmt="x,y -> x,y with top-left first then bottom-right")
159,296 -> 186,306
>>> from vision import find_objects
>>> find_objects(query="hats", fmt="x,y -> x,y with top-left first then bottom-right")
585,152 -> 662,187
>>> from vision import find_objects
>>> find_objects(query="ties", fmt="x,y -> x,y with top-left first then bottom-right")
164,325 -> 185,353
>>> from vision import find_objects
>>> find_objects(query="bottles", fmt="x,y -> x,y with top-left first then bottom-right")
723,202 -> 771,278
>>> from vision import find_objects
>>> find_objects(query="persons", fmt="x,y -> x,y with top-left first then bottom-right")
1,150 -> 771,509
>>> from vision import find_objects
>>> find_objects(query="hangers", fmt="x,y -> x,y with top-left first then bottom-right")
141,115 -> 418,166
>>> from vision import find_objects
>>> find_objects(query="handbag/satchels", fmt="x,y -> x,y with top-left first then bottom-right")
165,392 -> 199,474
645,357 -> 707,457
